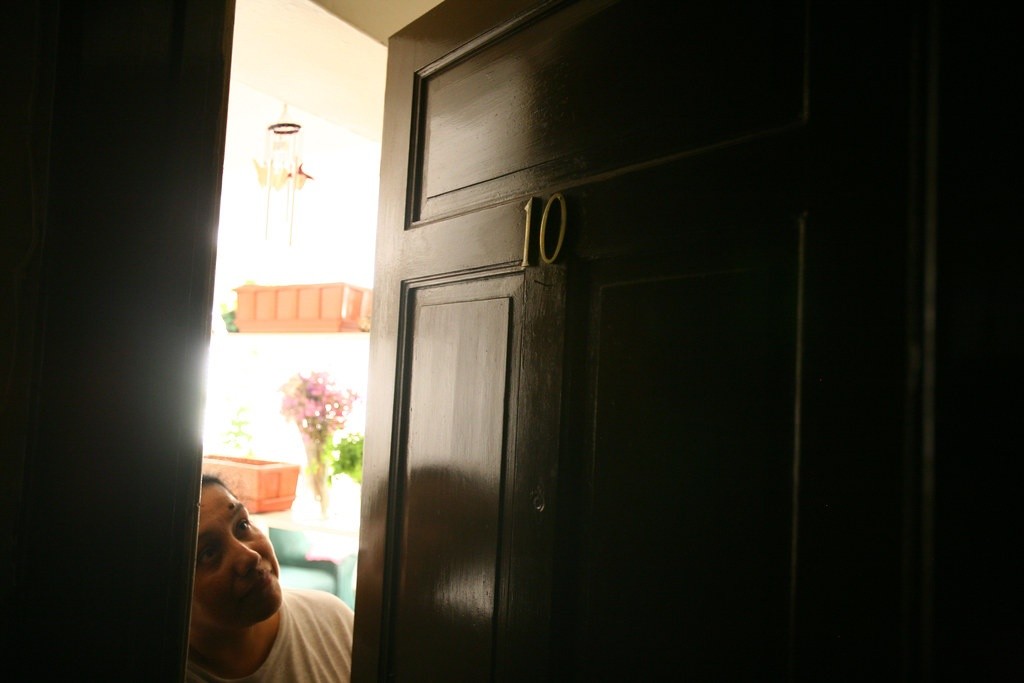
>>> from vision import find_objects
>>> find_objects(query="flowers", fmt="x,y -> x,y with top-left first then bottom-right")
281,370 -> 359,492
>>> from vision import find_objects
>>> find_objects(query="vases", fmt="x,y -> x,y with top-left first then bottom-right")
202,456 -> 301,514
233,283 -> 365,332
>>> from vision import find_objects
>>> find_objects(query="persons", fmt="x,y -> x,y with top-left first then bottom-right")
185,475 -> 355,683
